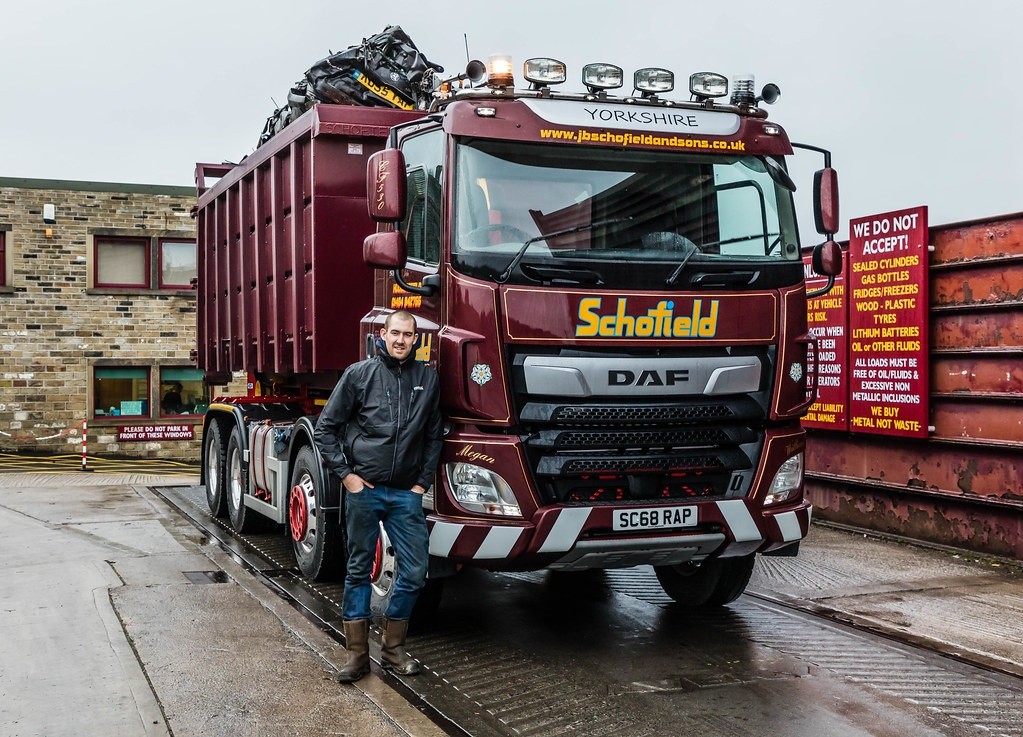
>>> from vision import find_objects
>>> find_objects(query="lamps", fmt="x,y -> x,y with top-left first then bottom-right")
43,204 -> 56,224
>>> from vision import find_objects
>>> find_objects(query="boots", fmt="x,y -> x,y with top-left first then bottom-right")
381,613 -> 421,674
338,619 -> 371,680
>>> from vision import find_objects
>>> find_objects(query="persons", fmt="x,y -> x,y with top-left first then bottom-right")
162,383 -> 183,414
313,310 -> 444,683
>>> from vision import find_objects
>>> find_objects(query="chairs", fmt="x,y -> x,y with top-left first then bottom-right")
464,182 -> 490,245
610,191 -> 679,248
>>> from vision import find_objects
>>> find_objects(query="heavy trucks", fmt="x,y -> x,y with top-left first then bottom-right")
190,54 -> 842,635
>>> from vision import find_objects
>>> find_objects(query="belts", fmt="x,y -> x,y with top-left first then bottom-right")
380,482 -> 411,490
